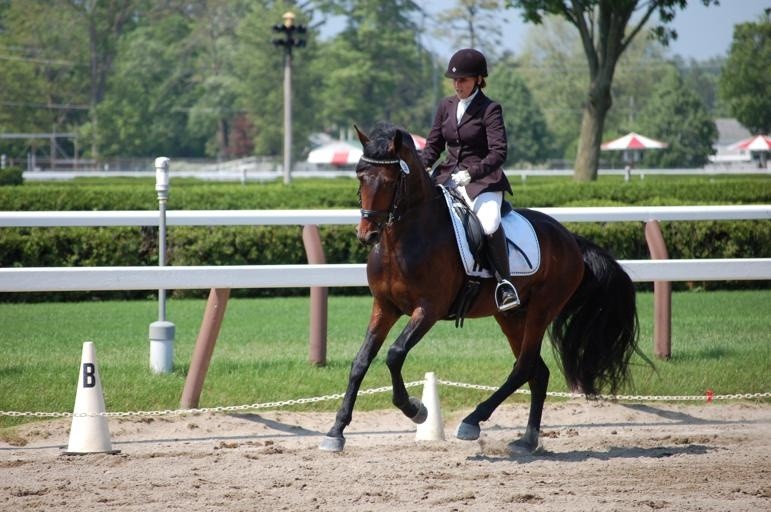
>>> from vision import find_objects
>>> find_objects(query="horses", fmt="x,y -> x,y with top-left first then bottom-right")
318,118 -> 655,454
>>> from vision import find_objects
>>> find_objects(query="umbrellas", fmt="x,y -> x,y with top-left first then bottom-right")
307,142 -> 362,171
729,134 -> 771,169
411,134 -> 426,151
600,132 -> 669,181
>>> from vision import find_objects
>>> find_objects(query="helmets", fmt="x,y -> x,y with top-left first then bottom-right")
445,48 -> 488,78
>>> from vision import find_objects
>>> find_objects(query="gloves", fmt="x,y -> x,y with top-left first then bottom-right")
451,170 -> 472,187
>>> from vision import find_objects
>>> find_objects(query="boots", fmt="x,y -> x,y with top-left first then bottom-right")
483,222 -> 517,304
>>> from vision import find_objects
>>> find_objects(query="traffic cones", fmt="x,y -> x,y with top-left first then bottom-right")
415,368 -> 446,443
57,338 -> 121,458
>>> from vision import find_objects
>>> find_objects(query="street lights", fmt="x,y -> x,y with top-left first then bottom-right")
271,10 -> 311,186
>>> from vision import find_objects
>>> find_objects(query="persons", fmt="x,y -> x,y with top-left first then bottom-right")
420,48 -> 517,303
